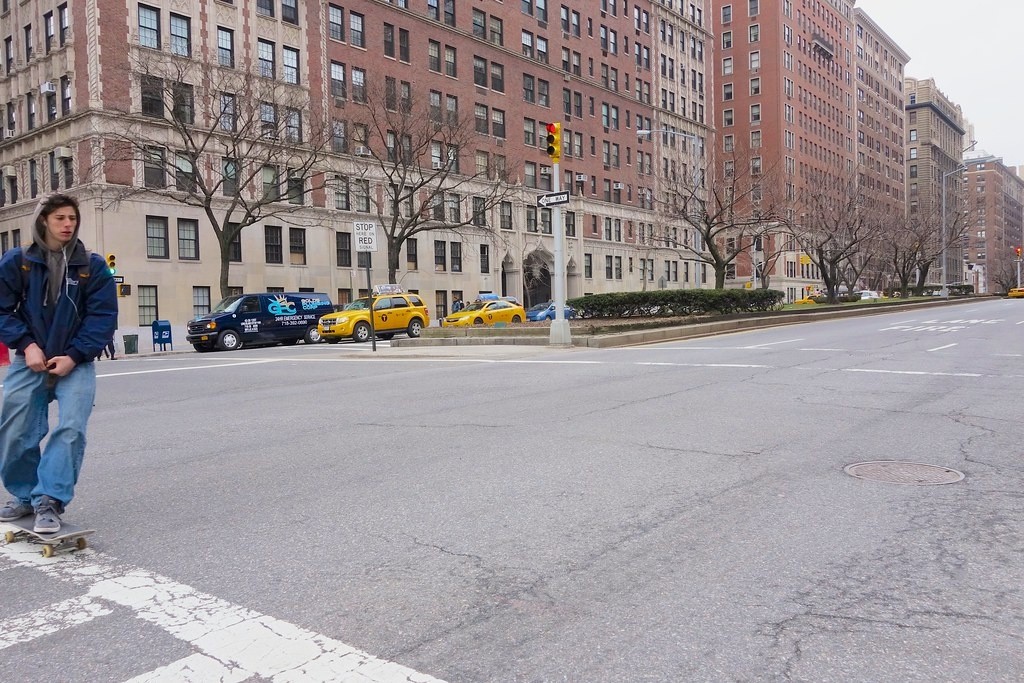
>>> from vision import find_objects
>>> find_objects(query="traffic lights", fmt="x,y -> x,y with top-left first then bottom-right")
1016,248 -> 1021,257
546,122 -> 561,157
117,283 -> 131,298
806,256 -> 811,265
106,253 -> 116,274
800,256 -> 805,264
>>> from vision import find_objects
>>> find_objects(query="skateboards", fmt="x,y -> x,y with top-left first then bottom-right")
4,513 -> 96,559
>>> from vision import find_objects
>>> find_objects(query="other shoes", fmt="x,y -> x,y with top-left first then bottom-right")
34,496 -> 62,533
98,358 -> 101,361
111,357 -> 117,360
0,500 -> 34,522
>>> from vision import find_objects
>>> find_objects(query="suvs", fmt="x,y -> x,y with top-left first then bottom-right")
500,296 -> 522,306
317,284 -> 430,344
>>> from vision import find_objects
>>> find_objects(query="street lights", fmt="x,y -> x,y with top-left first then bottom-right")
939,157 -> 1005,297
752,221 -> 779,289
634,129 -> 703,290
928,266 -> 943,284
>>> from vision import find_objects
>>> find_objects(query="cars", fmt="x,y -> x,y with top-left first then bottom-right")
526,301 -> 577,322
794,294 -> 822,304
441,293 -> 527,327
1008,287 -> 1024,298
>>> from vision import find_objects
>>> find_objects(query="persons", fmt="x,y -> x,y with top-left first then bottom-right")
97,336 -> 117,361
452,296 -> 481,313
0,192 -> 118,532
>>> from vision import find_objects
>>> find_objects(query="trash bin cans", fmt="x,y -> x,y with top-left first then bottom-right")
123,334 -> 138,354
152,320 -> 173,352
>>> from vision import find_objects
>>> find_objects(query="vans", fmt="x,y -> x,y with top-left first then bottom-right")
186,292 -> 335,353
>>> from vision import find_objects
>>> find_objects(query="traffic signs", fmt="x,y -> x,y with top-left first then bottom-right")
113,275 -> 124,283
750,277 -> 759,281
536,190 -> 571,208
